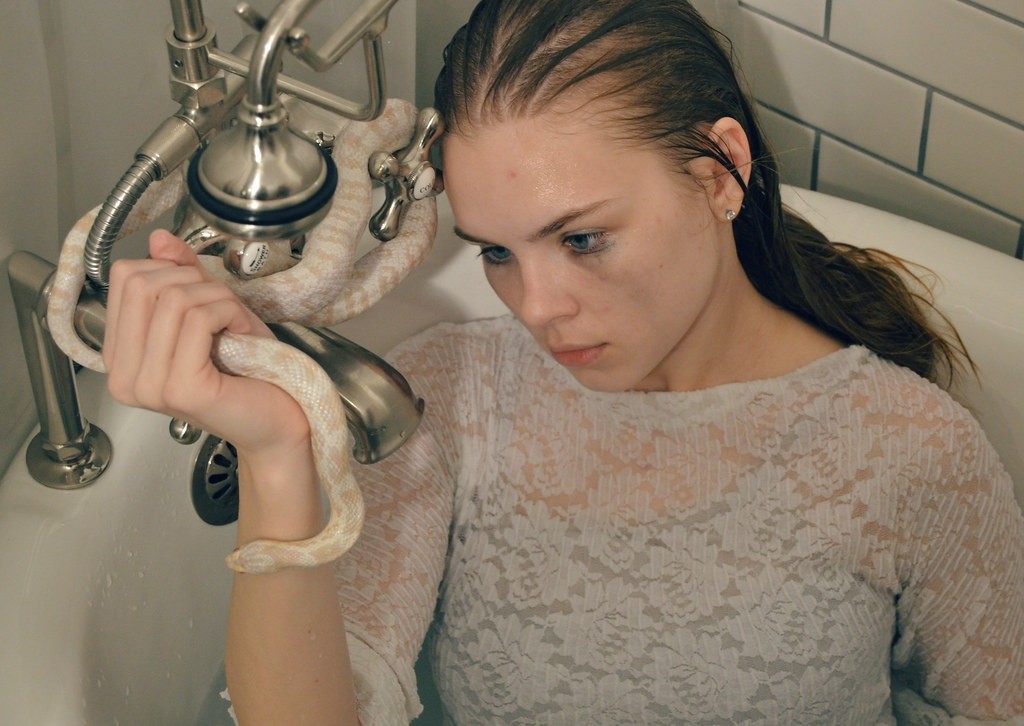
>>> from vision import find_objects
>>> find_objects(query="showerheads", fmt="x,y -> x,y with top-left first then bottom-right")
183,93 -> 339,242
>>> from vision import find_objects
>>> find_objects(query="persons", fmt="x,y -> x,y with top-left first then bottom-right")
102,0 -> 1024,726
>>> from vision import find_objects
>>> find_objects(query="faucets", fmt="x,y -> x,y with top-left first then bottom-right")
265,322 -> 425,465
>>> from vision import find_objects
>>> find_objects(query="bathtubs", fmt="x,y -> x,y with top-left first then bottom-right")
1,183 -> 1023,725
